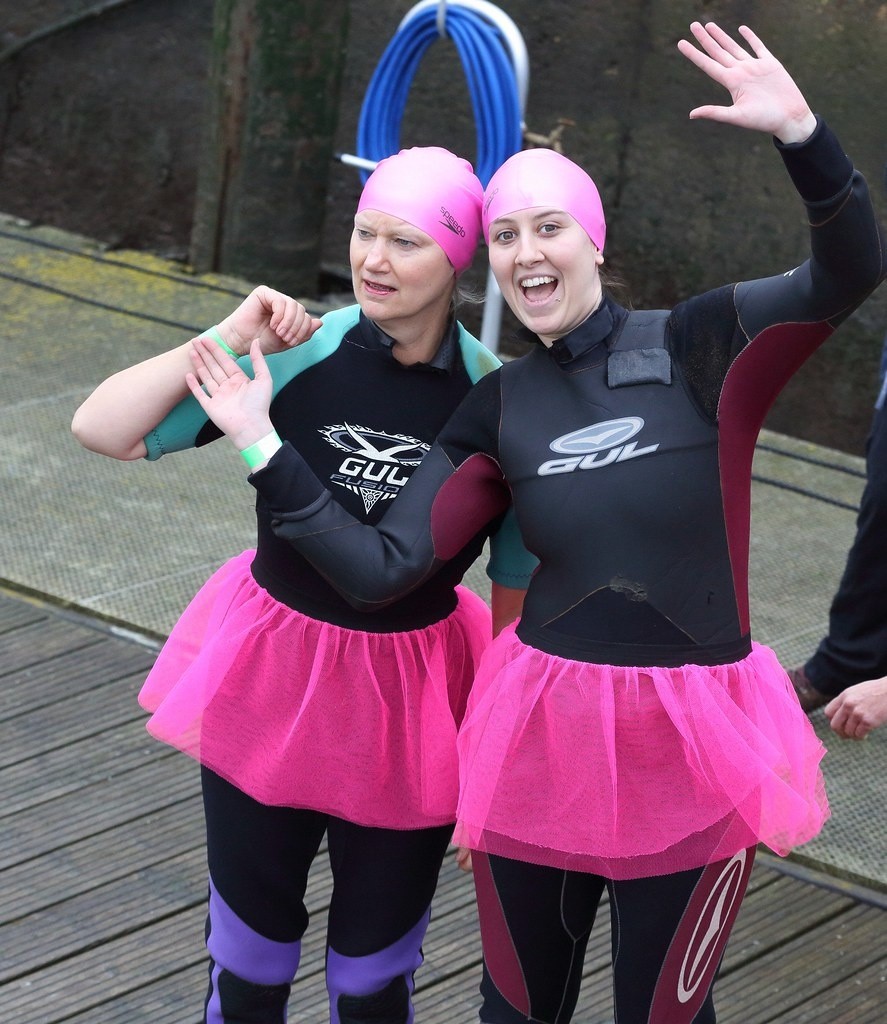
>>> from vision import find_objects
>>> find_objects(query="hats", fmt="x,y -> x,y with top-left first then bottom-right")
356,146 -> 484,278
481,149 -> 607,255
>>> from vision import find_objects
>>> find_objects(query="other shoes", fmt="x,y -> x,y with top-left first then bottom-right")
786,666 -> 832,714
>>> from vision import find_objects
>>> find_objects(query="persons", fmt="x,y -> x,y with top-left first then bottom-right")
182,21 -> 887,1024
68,147 -> 540,1024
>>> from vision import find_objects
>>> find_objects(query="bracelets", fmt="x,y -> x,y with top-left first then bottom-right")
240,429 -> 283,470
199,326 -> 240,365
